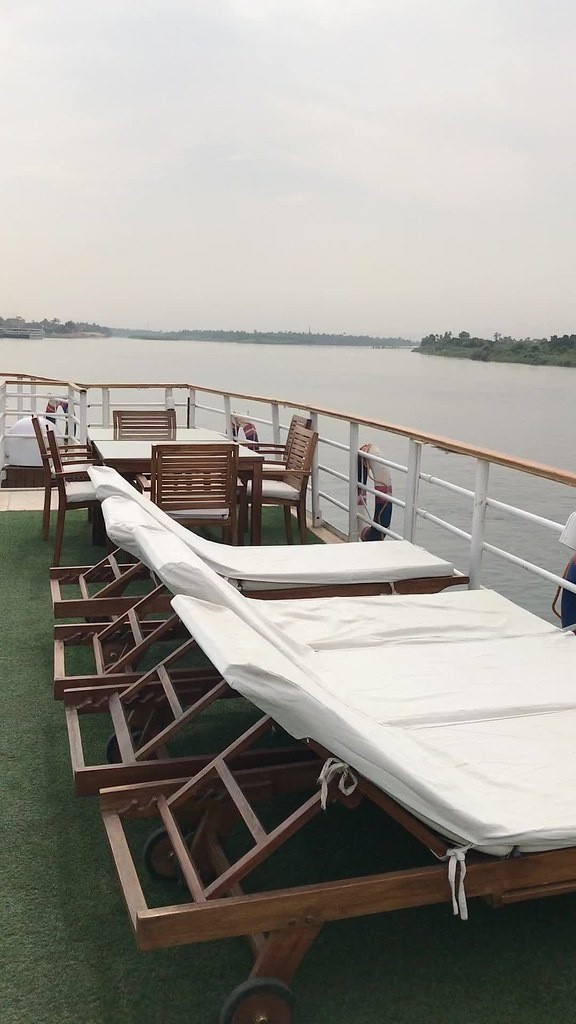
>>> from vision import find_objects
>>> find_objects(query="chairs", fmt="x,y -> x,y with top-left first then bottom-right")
33,393 -> 576,1024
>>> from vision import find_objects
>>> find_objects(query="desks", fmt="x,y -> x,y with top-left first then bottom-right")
85,427 -> 245,522
95,442 -> 264,555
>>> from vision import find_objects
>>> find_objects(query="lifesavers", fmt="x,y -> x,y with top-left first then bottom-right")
225,411 -> 259,450
46,398 -> 77,442
358,442 -> 393,542
